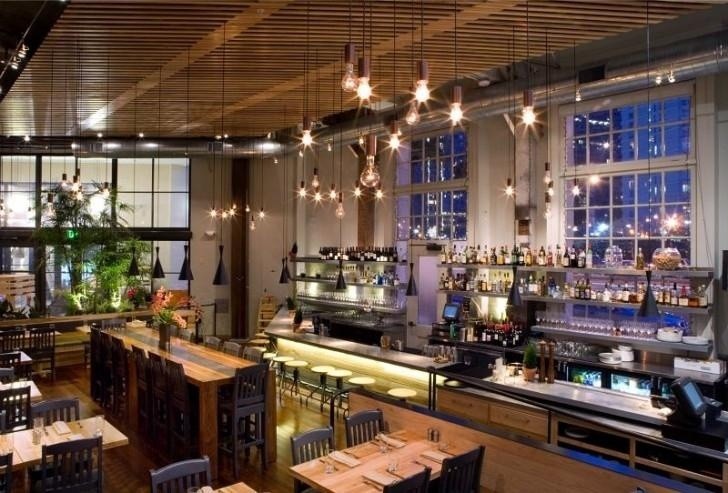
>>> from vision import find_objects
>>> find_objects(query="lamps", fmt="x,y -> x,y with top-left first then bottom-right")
357,1 -> 372,100
352,111 -> 389,202
186,19 -> 348,234
522,1 -> 535,126
342,1 -> 359,92
503,22 -> 675,237
1,39 -> 166,216
450,1 -> 463,120
416,1 -> 430,100
390,1 -> 401,149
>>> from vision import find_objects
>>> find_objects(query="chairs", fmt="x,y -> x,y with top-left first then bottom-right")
1,322 -> 269,492
288,407 -> 487,493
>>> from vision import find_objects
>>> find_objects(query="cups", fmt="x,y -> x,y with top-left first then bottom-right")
380,335 -> 390,352
324,448 -> 335,472
652,247 -> 681,270
492,356 -> 528,386
95,414 -> 105,437
33,416 -> 45,442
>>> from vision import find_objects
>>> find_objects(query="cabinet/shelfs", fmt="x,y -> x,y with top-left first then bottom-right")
433,262 -> 728,492
289,255 -> 400,332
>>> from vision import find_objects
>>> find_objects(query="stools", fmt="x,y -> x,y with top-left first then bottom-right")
320,370 -> 353,417
342,377 -> 376,421
306,366 -> 335,412
386,387 -> 417,409
232,332 -> 294,400
282,360 -> 313,404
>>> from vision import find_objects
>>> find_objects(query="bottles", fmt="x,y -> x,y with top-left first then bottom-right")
319,323 -> 329,339
636,248 -> 644,269
335,264 -> 400,286
320,244 -> 399,262
31,428 -> 41,444
438,244 -> 593,300
426,427 -> 439,442
448,316 -> 524,348
590,280 -> 708,307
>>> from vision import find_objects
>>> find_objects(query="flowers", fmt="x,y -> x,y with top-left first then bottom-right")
147,284 -> 203,327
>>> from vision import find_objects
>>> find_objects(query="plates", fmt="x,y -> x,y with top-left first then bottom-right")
658,325 -> 710,345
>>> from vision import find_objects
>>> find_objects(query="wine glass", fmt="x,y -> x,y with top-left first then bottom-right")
535,309 -> 657,339
